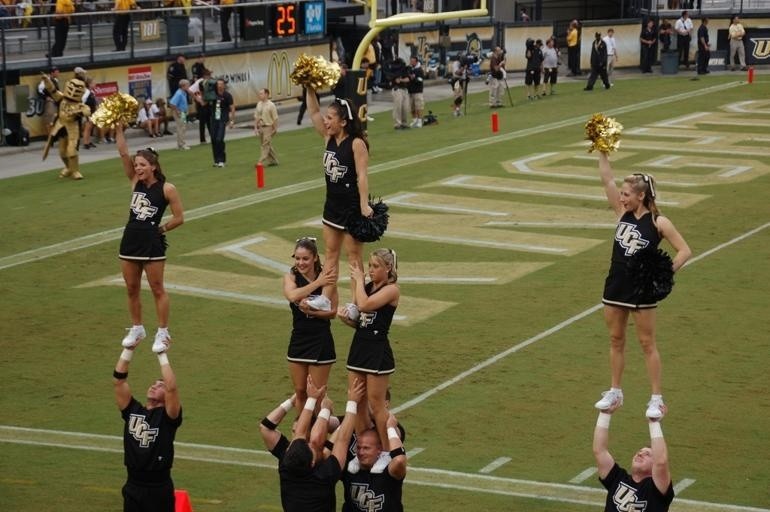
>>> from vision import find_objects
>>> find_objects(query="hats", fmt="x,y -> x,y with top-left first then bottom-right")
144,98 -> 153,105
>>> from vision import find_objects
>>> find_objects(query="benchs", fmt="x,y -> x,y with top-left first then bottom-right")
67,31 -> 87,49
0,35 -> 28,54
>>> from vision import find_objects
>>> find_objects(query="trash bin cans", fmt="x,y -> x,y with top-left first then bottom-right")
660,49 -> 680,74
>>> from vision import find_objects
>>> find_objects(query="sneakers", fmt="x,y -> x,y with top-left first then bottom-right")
369,450 -> 391,474
347,454 -> 360,475
150,326 -> 171,354
342,302 -> 359,321
594,388 -> 627,411
305,294 -> 332,311
372,62 -> 752,132
84,130 -> 280,168
644,393 -> 667,422
120,325 -> 147,348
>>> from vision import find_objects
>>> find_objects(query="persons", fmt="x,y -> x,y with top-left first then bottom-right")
0,1 -> 746,178
258,374 -> 407,512
115,120 -> 184,353
592,398 -> 675,512
595,139 -> 692,419
304,84 -> 374,323
337,247 -> 400,474
283,237 -> 337,416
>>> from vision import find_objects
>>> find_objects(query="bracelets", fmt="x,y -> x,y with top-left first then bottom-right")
119,348 -> 135,362
156,352 -> 170,366
647,422 -> 663,439
595,412 -> 612,429
